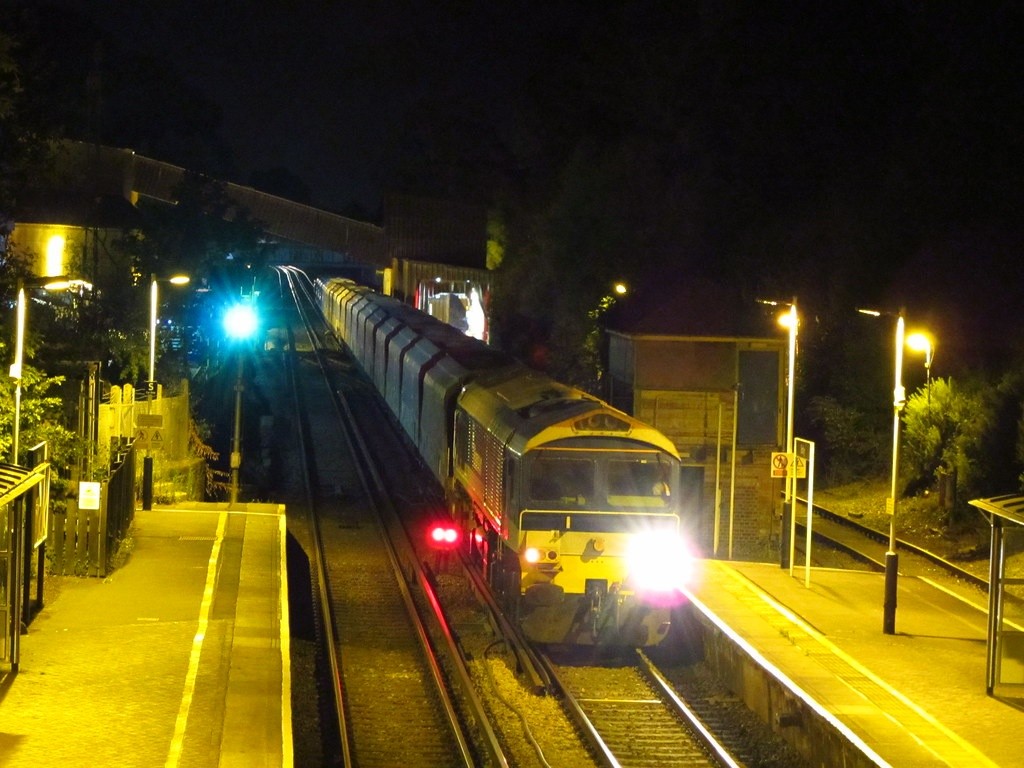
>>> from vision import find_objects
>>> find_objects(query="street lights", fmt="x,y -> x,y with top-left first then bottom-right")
754,292 -> 794,569
902,327 -> 935,413
224,303 -> 263,502
856,303 -> 905,634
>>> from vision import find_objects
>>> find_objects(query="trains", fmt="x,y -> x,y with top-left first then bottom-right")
311,271 -> 686,653
